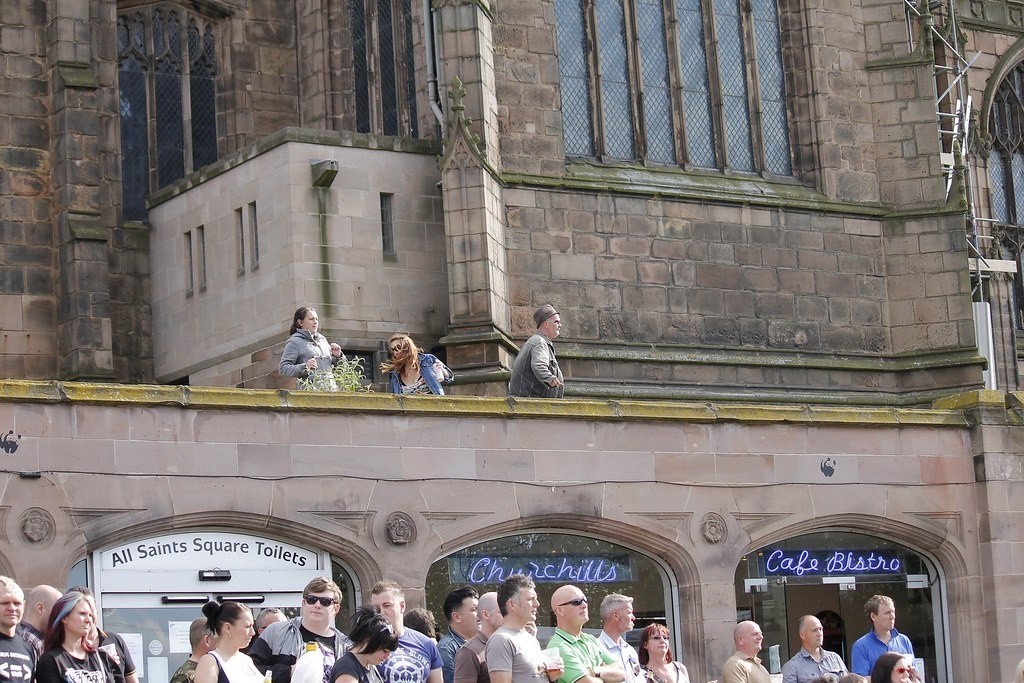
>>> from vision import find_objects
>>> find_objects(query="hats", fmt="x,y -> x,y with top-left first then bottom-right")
533,304 -> 560,330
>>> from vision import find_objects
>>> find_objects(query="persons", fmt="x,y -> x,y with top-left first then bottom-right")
722,620 -> 771,683
167,573 -> 644,683
379,333 -> 454,395
870,652 -> 921,683
0,575 -> 140,683
278,307 -> 347,391
806,672 -> 869,683
508,304 -> 564,399
850,594 -> 914,677
780,615 -> 849,683
639,623 -> 720,683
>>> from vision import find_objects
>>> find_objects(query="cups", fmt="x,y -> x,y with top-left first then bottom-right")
540,647 -> 560,670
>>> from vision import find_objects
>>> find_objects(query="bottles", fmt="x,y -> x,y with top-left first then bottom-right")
432,361 -> 444,383
309,356 -> 316,379
262,670 -> 272,683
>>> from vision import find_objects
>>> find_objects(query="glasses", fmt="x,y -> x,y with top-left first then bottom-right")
646,635 -> 670,641
305,594 -> 336,607
391,344 -> 402,352
558,597 -> 588,607
379,624 -> 393,634
893,668 -> 910,674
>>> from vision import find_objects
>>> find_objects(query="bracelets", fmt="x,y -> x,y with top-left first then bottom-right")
590,667 -> 595,676
